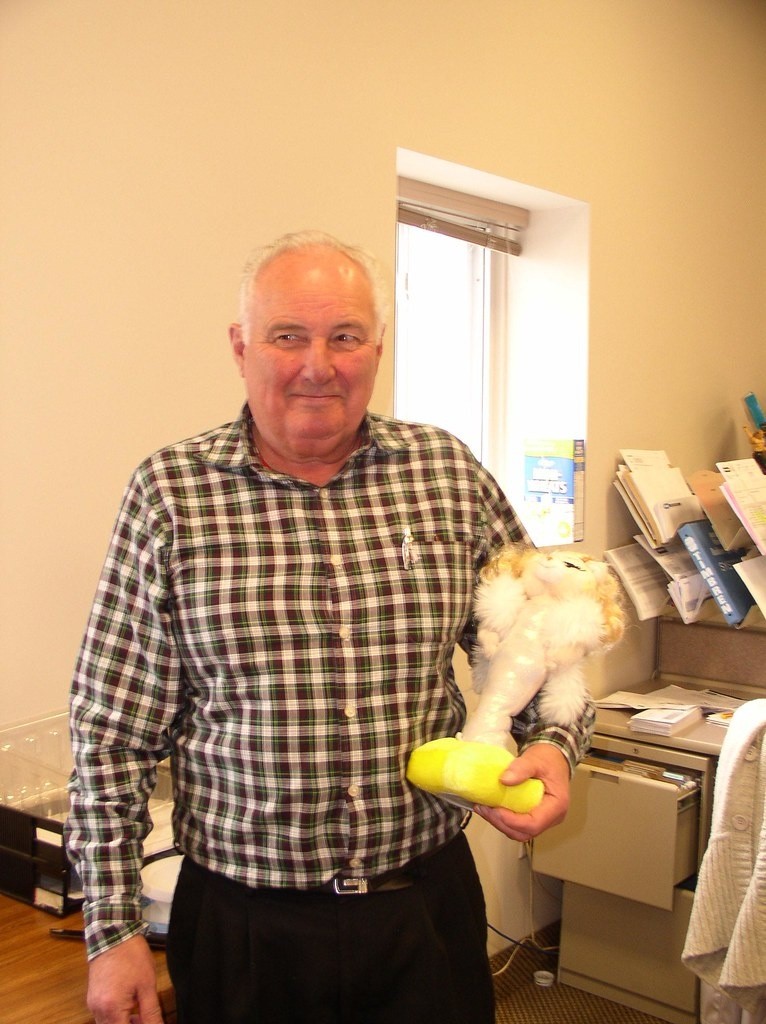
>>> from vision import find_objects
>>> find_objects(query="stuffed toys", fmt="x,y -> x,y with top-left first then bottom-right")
405,549 -> 627,811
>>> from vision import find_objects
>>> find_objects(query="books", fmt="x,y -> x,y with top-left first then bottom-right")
604,446 -> 766,625
596,681 -> 752,735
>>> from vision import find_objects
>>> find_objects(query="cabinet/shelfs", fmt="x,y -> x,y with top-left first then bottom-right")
531,682 -> 745,1023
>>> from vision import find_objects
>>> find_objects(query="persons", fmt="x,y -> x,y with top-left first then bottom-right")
65,232 -> 595,1024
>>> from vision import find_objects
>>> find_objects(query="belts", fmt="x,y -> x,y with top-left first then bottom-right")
308,840 -> 447,895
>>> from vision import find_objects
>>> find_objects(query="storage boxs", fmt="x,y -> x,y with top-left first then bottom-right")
523,435 -> 584,542
